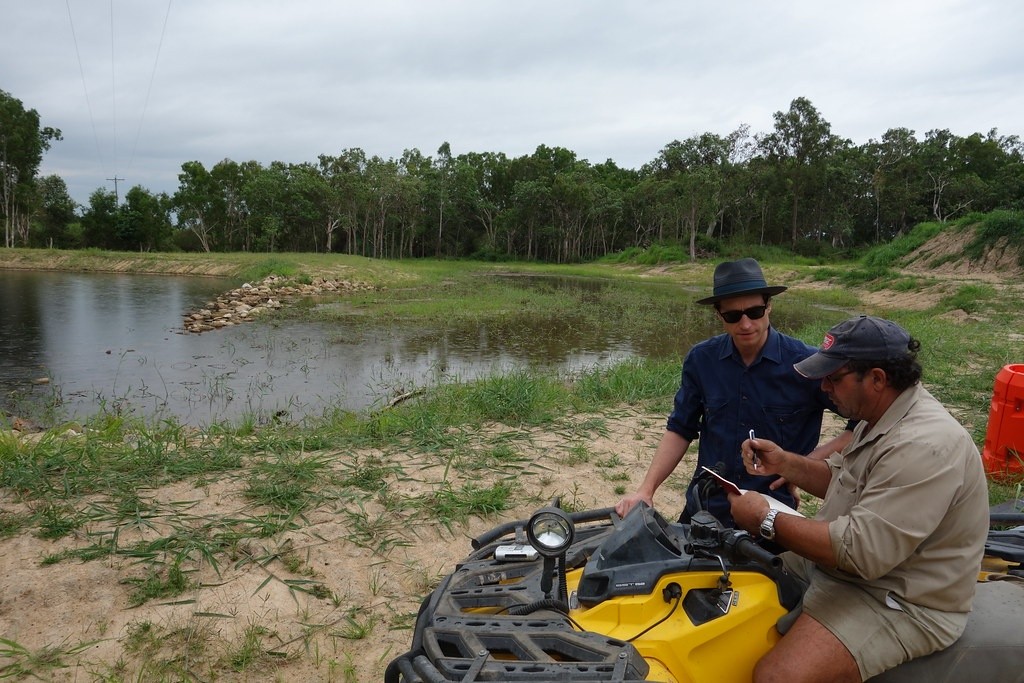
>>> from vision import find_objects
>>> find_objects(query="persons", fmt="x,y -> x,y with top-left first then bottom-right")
728,316 -> 990,683
615,257 -> 858,546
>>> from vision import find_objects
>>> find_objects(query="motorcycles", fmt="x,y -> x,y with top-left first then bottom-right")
384,460 -> 1023,683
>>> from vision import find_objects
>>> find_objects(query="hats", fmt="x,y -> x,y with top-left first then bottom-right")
695,257 -> 788,304
793,315 -> 915,380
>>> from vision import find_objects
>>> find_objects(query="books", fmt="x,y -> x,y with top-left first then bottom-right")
702,466 -> 806,518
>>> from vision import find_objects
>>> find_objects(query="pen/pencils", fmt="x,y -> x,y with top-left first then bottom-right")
748,427 -> 757,472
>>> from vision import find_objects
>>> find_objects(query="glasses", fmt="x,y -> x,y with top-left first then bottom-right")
714,299 -> 768,324
827,369 -> 857,385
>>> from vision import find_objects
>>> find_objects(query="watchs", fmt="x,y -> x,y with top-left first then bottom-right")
760,510 -> 779,540
